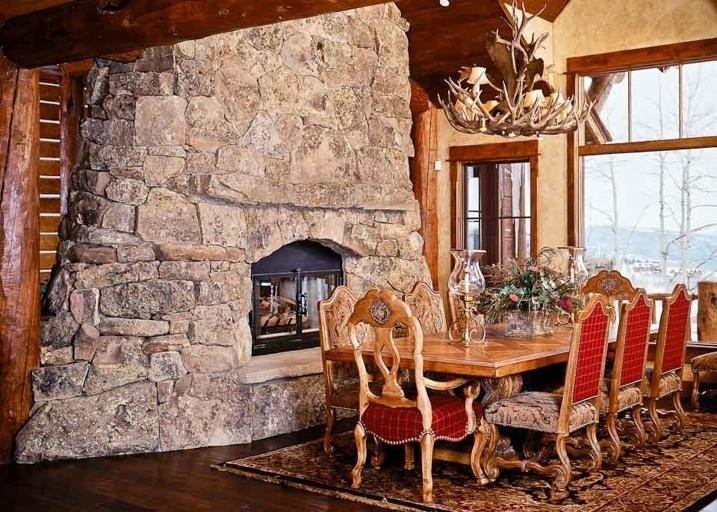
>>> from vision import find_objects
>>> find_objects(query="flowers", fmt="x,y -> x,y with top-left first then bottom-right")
460,257 -> 585,324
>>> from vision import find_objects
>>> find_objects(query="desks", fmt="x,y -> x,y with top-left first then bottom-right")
324,323 -> 659,479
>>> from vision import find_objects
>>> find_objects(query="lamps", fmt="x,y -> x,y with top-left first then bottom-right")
558,246 -> 589,326
449,250 -> 486,346
436,1 -> 594,136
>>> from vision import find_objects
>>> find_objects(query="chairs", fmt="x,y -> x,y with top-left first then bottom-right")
482,292 -> 613,493
348,287 -> 488,505
603,283 -> 697,444
580,270 -> 637,323
551,288 -> 654,465
318,284 -> 379,458
402,281 -> 448,337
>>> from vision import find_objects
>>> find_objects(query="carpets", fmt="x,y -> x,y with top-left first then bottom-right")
211,407 -> 717,510
